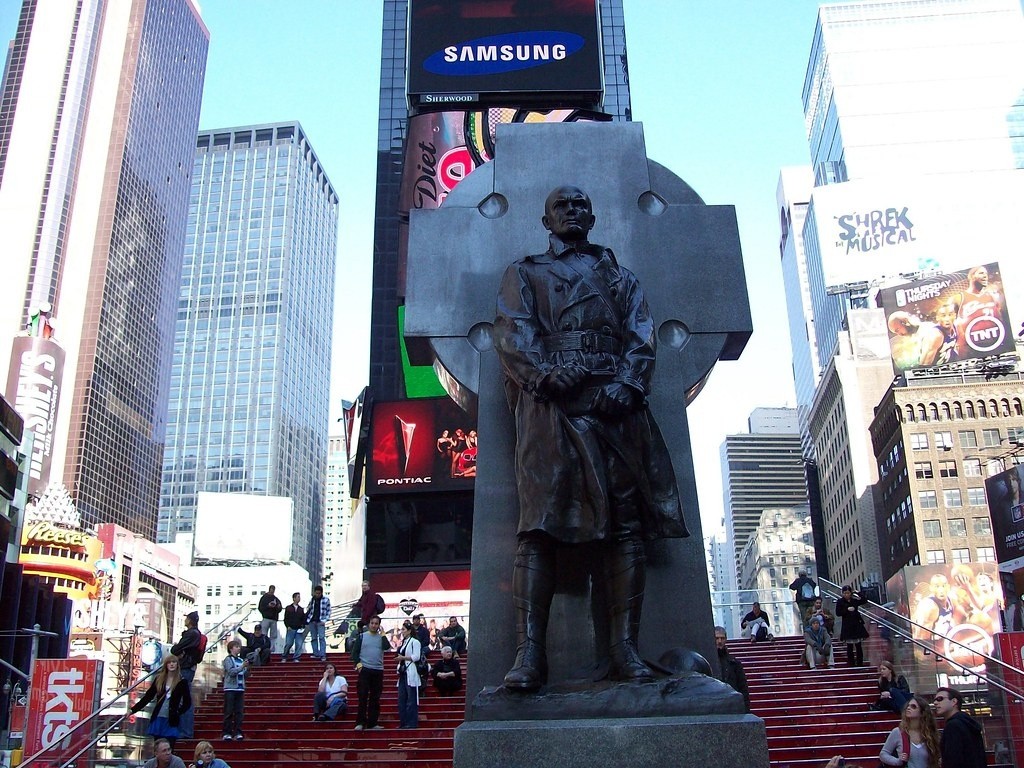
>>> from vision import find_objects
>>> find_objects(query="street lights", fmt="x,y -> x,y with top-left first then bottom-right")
3,679 -> 21,750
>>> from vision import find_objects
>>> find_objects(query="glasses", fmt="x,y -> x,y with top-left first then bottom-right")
905,704 -> 920,709
934,696 -> 949,701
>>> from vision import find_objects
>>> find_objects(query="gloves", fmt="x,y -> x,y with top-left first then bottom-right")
816,645 -> 831,656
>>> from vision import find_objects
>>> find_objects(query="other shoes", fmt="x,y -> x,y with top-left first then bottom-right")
281,659 -> 286,663
769,633 -> 775,641
235,734 -> 244,739
847,662 -> 867,667
314,714 -> 327,721
321,657 -> 326,661
222,734 -> 232,740
354,724 -> 364,731
452,650 -> 459,658
294,659 -> 299,662
396,724 -> 418,729
751,635 -> 756,642
372,725 -> 385,729
310,655 -> 320,658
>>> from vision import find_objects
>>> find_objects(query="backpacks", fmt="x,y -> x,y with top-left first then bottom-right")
796,577 -> 814,599
374,593 -> 386,614
411,640 -> 429,675
185,627 -> 208,664
876,727 -> 910,768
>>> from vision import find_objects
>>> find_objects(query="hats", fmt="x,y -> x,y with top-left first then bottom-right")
809,617 -> 819,626
798,569 -> 807,577
184,611 -> 199,623
254,624 -> 262,630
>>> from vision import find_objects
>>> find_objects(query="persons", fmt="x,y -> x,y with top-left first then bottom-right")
913,573 -> 1003,641
171,610 -> 201,739
312,663 -> 349,721
412,615 -> 466,697
880,686 -> 986,768
350,580 -> 391,731
491,184 -> 693,689
825,754 -> 863,768
141,737 -> 232,768
237,585 -> 331,666
788,570 -> 870,670
394,622 -> 426,729
221,640 -> 249,741
741,602 -> 776,642
877,660 -> 915,714
122,655 -> 192,758
998,467 -> 1024,531
715,626 -> 750,713
888,266 -> 1003,365
436,429 -> 477,479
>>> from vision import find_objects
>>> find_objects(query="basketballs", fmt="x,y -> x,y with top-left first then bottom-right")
951,564 -> 974,585
968,612 -> 992,631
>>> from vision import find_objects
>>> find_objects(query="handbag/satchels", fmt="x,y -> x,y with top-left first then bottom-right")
246,649 -> 260,666
756,627 -> 767,641
